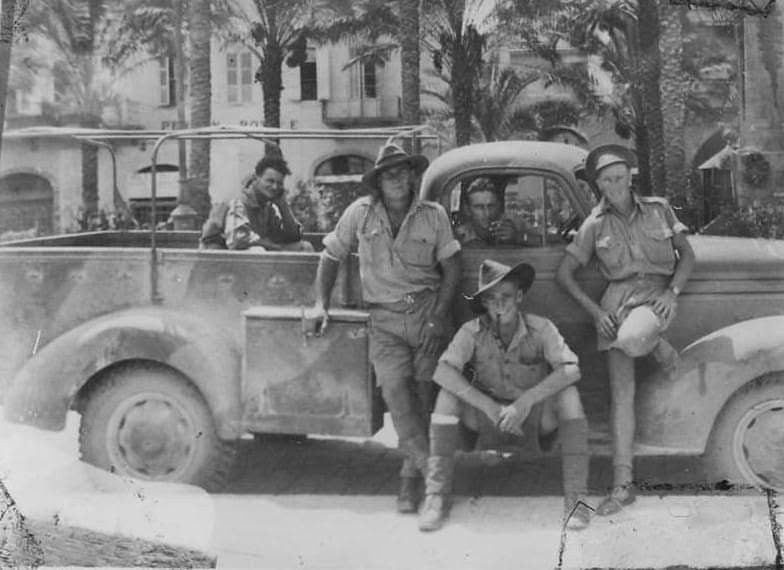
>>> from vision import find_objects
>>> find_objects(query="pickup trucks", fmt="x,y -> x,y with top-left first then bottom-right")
0,122 -> 784,496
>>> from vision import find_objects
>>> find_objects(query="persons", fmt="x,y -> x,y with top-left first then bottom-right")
302,145 -> 463,514
556,143 -> 696,516
199,155 -> 317,252
451,176 -> 559,246
420,259 -> 595,531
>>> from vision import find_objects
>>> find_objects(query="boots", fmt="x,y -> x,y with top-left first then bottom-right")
561,455 -> 589,528
418,456 -> 452,528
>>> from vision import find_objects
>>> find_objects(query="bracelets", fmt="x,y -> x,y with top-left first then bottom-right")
522,231 -> 529,245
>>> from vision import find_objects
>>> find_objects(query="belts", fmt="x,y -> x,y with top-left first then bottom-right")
376,289 -> 434,311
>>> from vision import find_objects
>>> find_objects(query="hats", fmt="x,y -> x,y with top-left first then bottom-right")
362,144 -> 429,196
585,145 -> 634,181
539,125 -> 589,144
461,259 -> 535,302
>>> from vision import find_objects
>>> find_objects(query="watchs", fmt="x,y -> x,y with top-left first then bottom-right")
668,285 -> 681,295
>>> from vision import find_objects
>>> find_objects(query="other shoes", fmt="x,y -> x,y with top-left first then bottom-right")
597,484 -> 635,515
399,479 -> 419,512
653,338 -> 679,381
